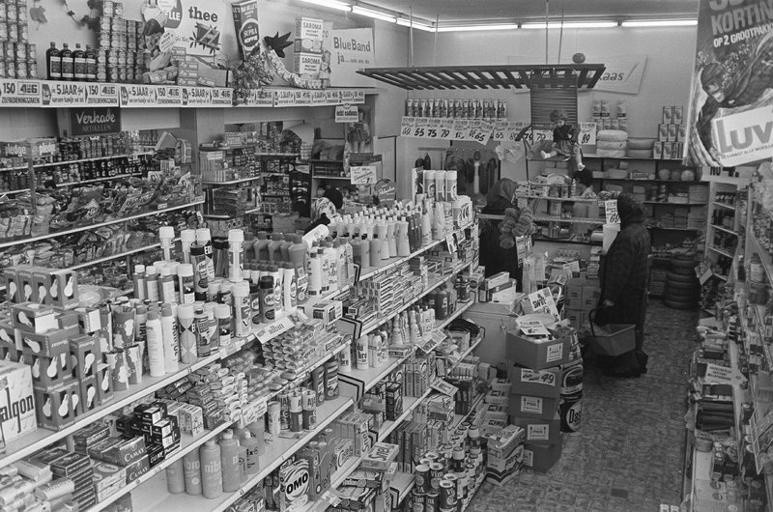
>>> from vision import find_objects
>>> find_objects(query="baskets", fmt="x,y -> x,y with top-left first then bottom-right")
585,304 -> 637,360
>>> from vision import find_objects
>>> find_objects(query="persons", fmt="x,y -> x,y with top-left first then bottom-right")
537,106 -> 587,173
590,189 -> 650,378
473,176 -> 534,293
302,186 -> 348,234
346,106 -> 372,154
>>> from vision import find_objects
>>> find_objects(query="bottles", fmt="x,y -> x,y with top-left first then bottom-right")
166,431 -> 259,499
46,41 -> 96,82
133,170 -> 473,378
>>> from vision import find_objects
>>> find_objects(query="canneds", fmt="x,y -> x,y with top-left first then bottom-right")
404,422 -> 483,512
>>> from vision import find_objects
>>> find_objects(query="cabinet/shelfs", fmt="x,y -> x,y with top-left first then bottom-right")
0,77 -> 773,511
